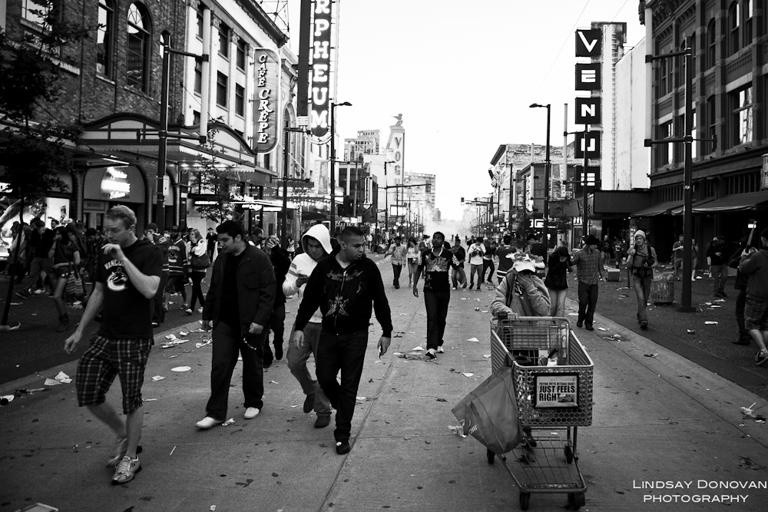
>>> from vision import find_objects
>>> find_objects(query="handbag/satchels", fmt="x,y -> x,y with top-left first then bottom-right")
452,364 -> 521,455
190,251 -> 210,270
64,273 -> 84,298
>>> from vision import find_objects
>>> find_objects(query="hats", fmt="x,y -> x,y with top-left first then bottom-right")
513,259 -> 536,273
634,230 -> 645,238
585,234 -> 598,246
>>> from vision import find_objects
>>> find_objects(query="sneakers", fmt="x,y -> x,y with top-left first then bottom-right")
424,346 -> 443,358
335,439 -> 350,454
754,350 -> 768,365
111,456 -> 141,484
244,406 -> 259,418
105,435 -> 129,468
303,394 -> 314,413
195,417 -> 225,429
314,415 -> 330,428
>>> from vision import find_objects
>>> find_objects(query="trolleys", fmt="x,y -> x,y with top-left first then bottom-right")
486,317 -> 594,512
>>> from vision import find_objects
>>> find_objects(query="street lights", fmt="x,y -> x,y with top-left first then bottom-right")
501,162 -> 513,247
385,160 -> 396,242
330,102 -> 352,237
530,103 -> 551,270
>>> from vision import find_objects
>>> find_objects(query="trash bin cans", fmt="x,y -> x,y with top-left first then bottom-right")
650,264 -> 675,306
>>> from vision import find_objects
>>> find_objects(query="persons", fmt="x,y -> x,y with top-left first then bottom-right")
1,204 -> 767,486
